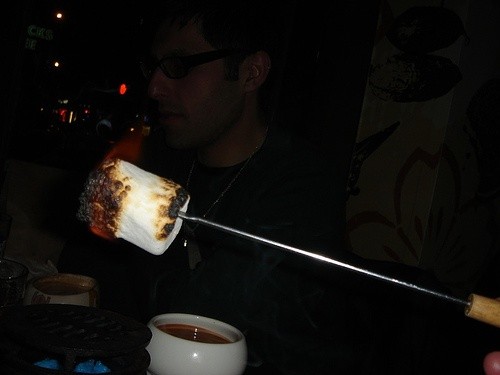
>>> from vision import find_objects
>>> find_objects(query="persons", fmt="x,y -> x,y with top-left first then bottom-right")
0,0 -> 381,375
482,350 -> 500,375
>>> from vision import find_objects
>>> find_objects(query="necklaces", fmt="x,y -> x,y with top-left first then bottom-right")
180,126 -> 269,247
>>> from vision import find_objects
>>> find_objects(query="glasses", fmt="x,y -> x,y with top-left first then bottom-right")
137,47 -> 240,78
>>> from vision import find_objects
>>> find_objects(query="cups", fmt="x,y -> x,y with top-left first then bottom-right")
0,258 -> 28,310
24,274 -> 97,310
145,314 -> 248,375
